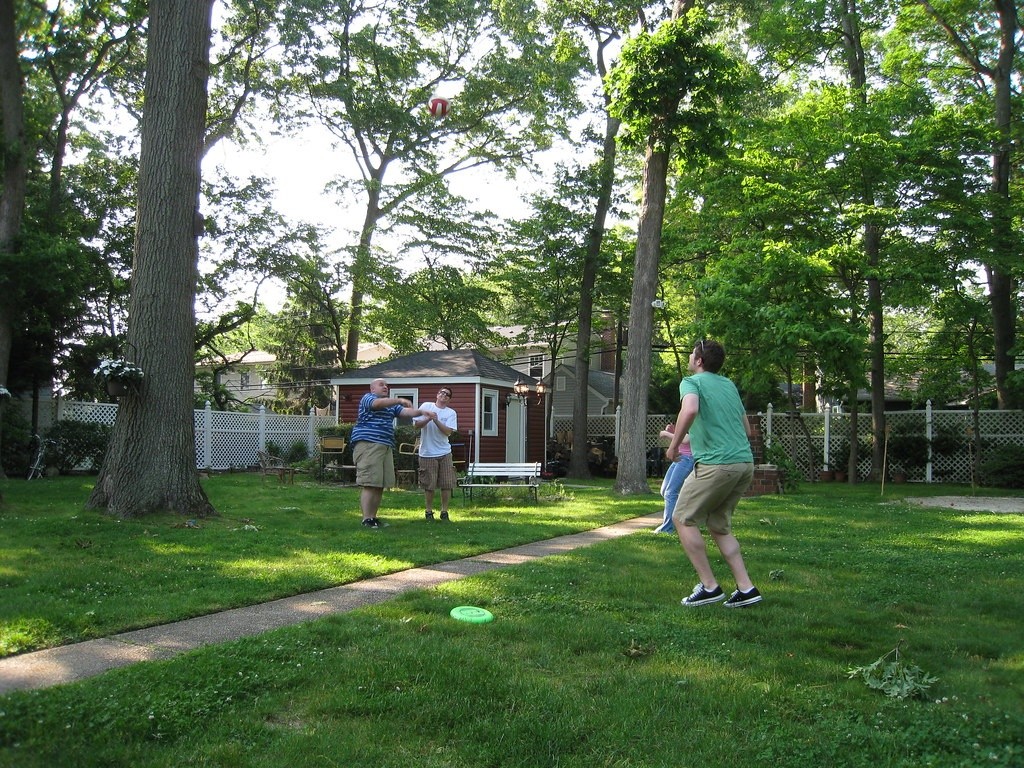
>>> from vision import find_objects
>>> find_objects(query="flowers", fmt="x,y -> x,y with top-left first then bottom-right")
105,356 -> 145,381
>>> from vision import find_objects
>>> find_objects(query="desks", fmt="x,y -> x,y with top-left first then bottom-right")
327,465 -> 357,485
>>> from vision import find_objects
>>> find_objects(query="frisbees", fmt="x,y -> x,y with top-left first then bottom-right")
450,605 -> 494,624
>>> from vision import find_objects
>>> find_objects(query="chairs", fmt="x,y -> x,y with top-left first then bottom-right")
647,446 -> 672,479
256,450 -> 294,484
399,437 -> 422,470
316,436 -> 347,486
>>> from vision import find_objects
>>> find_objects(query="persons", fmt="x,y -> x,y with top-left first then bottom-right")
350,379 -> 435,529
652,338 -> 763,608
413,388 -> 457,521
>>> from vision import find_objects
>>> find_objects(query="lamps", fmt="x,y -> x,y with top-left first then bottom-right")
519,376 -> 530,405
513,375 -> 521,399
536,378 -> 547,406
505,392 -> 512,407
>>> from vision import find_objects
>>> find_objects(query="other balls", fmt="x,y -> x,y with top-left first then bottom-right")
427,94 -> 451,117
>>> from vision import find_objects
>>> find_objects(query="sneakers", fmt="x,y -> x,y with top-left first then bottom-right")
680,582 -> 725,606
723,584 -> 763,607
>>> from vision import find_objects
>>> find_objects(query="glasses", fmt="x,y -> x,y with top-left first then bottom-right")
699,340 -> 705,353
438,391 -> 451,397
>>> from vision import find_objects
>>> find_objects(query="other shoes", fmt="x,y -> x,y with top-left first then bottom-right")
361,517 -> 383,529
425,510 -> 434,519
440,511 -> 450,522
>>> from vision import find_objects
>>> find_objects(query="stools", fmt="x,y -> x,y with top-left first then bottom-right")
397,469 -> 416,491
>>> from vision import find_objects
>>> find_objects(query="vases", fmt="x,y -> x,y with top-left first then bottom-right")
819,471 -> 833,482
106,381 -> 129,397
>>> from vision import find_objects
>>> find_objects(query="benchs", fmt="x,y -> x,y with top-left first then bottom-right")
459,460 -> 542,507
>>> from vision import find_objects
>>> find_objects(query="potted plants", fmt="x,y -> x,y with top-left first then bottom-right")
887,421 -> 934,484
827,438 -> 850,482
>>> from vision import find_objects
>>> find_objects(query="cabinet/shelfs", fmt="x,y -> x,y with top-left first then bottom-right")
450,442 -> 467,473
